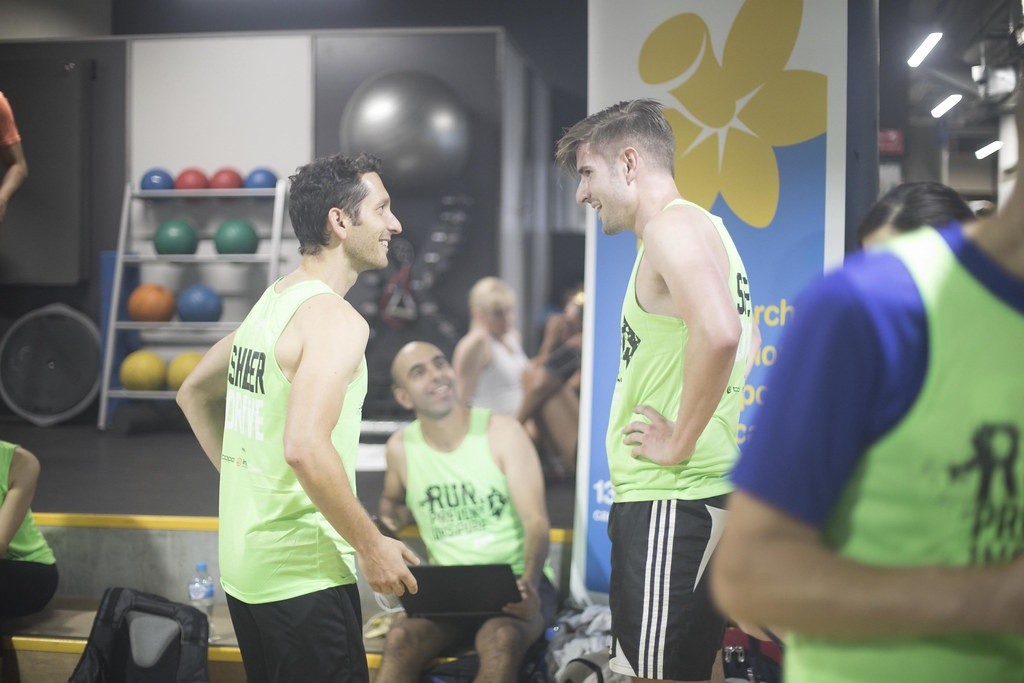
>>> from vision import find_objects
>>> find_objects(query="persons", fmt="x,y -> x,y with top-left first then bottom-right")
451,272 -> 583,472
554,98 -> 754,683
0,439 -> 61,683
0,88 -> 28,227
706,58 -> 1024,683
856,176 -> 966,257
174,149 -> 422,683
378,338 -> 554,683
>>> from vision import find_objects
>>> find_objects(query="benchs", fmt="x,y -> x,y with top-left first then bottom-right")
11,511 -> 575,683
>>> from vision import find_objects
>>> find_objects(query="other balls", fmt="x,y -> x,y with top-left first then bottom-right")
214,218 -> 259,264
168,351 -> 204,391
142,168 -> 279,204
154,219 -> 198,264
127,282 -> 176,322
178,285 -> 222,322
120,349 -> 167,390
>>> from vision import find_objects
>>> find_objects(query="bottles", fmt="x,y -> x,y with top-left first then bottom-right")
187,564 -> 216,641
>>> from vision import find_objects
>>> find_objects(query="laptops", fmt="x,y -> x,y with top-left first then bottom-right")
399,563 -> 523,619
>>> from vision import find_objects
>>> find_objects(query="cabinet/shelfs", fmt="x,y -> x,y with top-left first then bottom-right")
98,177 -> 287,429
